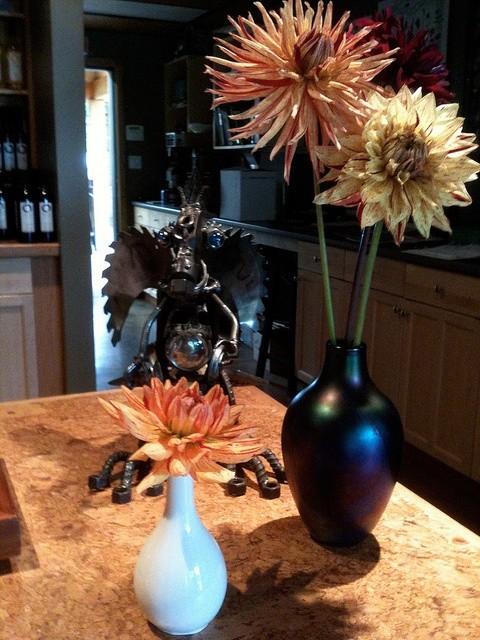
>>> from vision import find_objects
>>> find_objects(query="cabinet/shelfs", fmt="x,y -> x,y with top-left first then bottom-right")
0,0 -> 64,406
404,264 -> 480,485
210,36 -> 277,149
162,52 -> 210,133
133,206 -> 169,298
296,240 -> 404,437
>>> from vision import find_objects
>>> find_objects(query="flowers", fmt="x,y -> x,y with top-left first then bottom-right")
96,376 -> 267,498
203,0 -> 480,349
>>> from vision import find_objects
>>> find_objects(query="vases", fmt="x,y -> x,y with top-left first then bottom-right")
280,339 -> 405,550
134,474 -> 226,635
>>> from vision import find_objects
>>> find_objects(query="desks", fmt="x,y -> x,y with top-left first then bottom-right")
0,378 -> 480,640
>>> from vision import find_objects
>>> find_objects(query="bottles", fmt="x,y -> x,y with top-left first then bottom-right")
4,33 -> 25,90
0,185 -> 12,240
0,129 -> 16,177
16,130 -> 31,173
16,168 -> 39,243
36,169 -> 58,241
